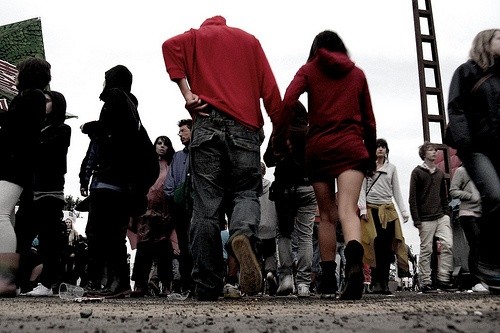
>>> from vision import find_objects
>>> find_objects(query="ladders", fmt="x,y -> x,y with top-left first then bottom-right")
412,0 -> 454,218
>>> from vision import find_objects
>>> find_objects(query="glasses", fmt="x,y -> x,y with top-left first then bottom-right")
177,128 -> 190,135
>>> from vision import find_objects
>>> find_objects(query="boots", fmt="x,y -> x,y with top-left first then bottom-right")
96,277 -> 130,298
83,274 -> 109,296
318,261 -> 338,294
0,252 -> 20,298
341,240 -> 365,299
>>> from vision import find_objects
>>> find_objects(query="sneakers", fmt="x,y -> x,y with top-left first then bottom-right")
297,285 -> 310,297
276,274 -> 294,296
472,283 -> 488,292
27,284 -> 53,296
422,285 -> 438,293
441,284 -> 458,291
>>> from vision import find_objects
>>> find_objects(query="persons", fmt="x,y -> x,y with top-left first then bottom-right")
388,237 -> 422,292
272,31 -> 377,299
162,16 -> 293,301
78,65 -> 141,297
15,90 -> 71,296
449,29 -> 500,296
409,143 -> 458,294
58,140 -> 110,290
362,258 -> 381,293
163,119 -> 196,298
130,135 -> 176,297
126,218 -> 181,296
21,234 -> 46,293
449,161 -> 490,293
0,58 -> 51,298
220,220 -> 243,298
254,161 -> 346,295
264,100 -> 317,296
357,139 -> 408,295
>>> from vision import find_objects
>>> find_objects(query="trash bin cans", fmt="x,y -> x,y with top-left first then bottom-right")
449,198 -> 473,282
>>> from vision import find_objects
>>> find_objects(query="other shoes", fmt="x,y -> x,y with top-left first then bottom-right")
372,283 -> 382,292
131,286 -> 144,297
384,288 -> 392,295
395,287 -> 402,292
405,287 -> 410,292
195,287 -> 220,301
232,236 -> 263,293
267,273 -> 278,296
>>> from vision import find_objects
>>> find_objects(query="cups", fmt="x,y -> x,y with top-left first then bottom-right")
59,283 -> 84,299
